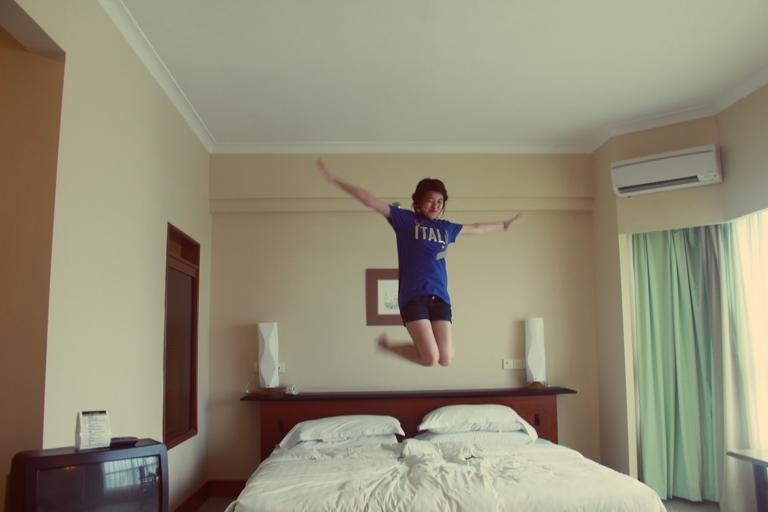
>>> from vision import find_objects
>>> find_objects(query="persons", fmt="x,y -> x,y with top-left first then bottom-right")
316,159 -> 522,367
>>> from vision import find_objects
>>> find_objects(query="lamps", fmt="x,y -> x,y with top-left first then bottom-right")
524,317 -> 548,391
257,322 -> 283,397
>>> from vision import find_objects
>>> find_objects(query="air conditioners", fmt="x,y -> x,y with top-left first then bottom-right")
609,144 -> 724,198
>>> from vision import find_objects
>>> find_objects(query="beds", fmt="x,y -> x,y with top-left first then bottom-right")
223,385 -> 671,512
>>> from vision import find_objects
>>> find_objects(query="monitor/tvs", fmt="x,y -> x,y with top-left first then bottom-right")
6,437 -> 169,512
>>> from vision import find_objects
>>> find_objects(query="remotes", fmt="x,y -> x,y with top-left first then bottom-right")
110,437 -> 139,447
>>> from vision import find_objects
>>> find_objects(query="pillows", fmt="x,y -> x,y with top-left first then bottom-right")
276,404 -> 539,461
